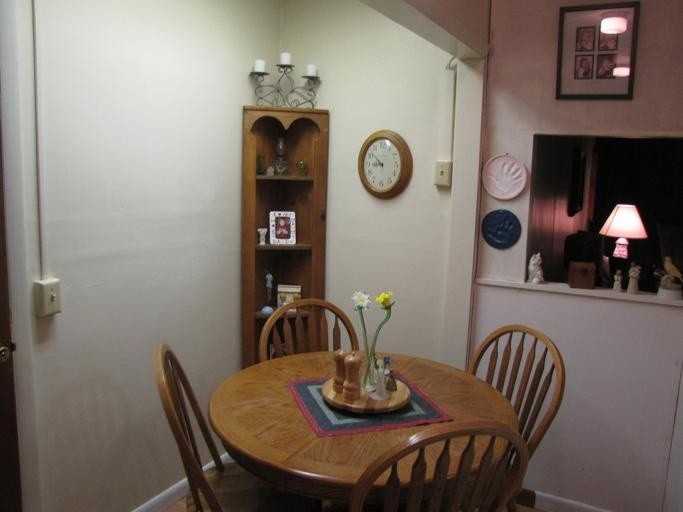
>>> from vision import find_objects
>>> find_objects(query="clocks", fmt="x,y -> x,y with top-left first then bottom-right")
358,130 -> 413,199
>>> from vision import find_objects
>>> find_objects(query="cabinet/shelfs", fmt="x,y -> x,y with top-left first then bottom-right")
241,105 -> 329,369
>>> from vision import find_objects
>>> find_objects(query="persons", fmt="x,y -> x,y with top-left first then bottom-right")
576,58 -> 593,79
599,30 -> 616,49
577,30 -> 592,51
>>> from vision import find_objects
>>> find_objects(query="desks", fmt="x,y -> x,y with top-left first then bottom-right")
209,350 -> 520,510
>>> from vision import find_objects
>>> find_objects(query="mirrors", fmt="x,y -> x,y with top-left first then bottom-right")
525,134 -> 683,301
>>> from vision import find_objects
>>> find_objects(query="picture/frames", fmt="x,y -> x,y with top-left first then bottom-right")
555,3 -> 639,100
269,211 -> 297,246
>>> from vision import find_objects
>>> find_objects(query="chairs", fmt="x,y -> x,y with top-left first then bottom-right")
468,324 -> 565,459
258,298 -> 359,362
153,340 -> 324,511
347,421 -> 530,510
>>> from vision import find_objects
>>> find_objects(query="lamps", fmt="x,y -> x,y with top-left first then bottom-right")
600,11 -> 630,34
599,204 -> 648,258
613,56 -> 630,77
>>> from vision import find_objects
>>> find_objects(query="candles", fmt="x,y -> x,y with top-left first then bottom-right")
280,53 -> 291,64
254,59 -> 265,72
306,65 -> 317,76
